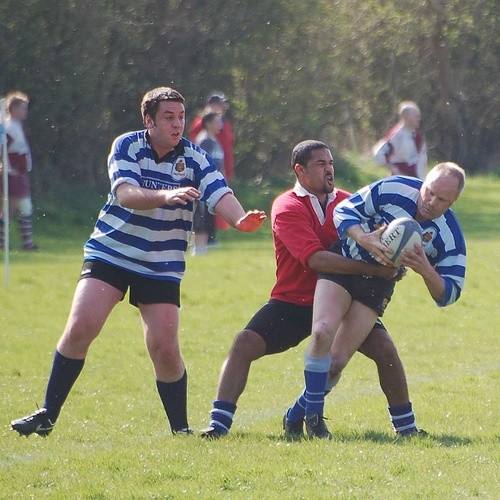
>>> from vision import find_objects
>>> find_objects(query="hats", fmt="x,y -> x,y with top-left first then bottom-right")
208,95 -> 229,104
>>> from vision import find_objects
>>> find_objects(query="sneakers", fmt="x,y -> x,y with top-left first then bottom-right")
10,404 -> 58,438
282,408 -> 304,443
305,413 -> 332,442
171,428 -> 195,438
200,427 -> 222,439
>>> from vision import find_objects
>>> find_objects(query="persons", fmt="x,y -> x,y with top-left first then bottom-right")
283,162 -> 466,439
373,100 -> 428,182
0,91 -> 40,251
201,139 -> 433,439
11,86 -> 267,438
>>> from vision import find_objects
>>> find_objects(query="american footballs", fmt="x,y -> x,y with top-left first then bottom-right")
375,219 -> 422,266
